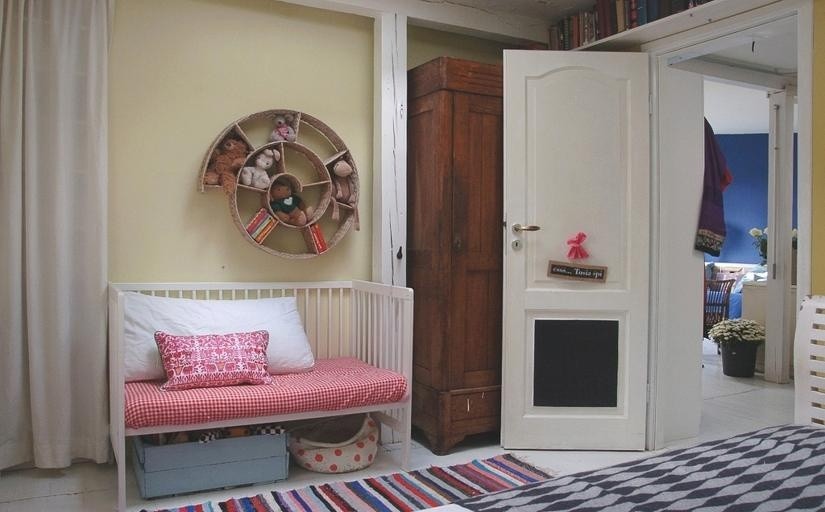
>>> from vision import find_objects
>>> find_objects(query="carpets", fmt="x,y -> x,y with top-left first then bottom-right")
168,450 -> 557,512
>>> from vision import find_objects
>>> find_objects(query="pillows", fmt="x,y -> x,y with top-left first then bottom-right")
121,290 -> 317,394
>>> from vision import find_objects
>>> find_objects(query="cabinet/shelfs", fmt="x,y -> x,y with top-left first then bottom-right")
406,56 -> 504,456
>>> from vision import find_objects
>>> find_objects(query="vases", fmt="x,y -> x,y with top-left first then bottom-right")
721,342 -> 759,377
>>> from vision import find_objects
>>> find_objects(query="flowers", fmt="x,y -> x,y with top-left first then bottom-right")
708,318 -> 766,345
750,225 -> 797,264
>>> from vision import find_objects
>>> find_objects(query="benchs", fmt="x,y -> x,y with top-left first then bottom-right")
106,279 -> 416,511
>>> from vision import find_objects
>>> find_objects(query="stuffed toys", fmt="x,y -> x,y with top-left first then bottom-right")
204,112 -> 361,233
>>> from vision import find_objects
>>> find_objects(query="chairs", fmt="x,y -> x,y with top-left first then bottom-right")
704,279 -> 736,353
